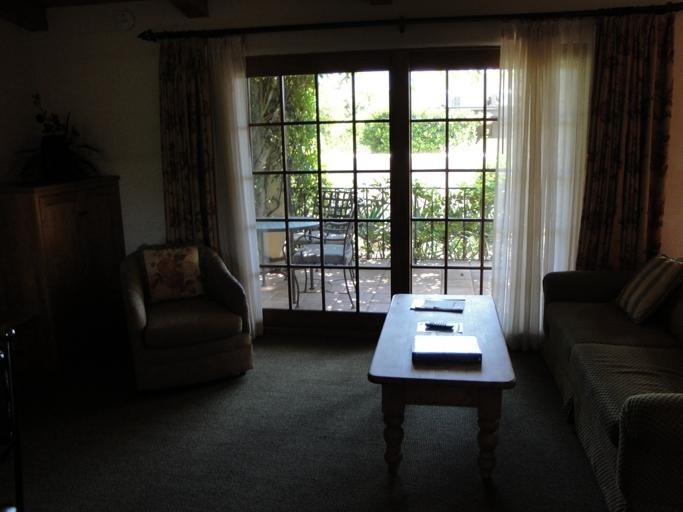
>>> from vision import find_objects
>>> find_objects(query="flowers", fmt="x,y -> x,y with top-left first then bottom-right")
15,92 -> 101,176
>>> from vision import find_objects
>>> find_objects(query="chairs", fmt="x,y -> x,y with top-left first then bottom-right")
119,244 -> 253,391
282,191 -> 360,309
0,328 -> 24,512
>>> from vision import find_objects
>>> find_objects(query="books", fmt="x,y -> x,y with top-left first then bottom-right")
409,296 -> 465,313
410,334 -> 482,366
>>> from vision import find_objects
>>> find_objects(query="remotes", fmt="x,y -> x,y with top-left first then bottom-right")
425,320 -> 453,328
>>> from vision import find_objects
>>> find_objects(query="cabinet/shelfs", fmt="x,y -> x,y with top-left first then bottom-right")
0,174 -> 124,384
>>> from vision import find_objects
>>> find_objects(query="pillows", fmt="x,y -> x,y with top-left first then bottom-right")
615,254 -> 683,326
138,244 -> 208,305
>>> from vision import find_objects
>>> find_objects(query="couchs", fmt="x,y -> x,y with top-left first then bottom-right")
543,256 -> 683,512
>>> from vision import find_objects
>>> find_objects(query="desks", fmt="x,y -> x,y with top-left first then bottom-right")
256,217 -> 321,303
